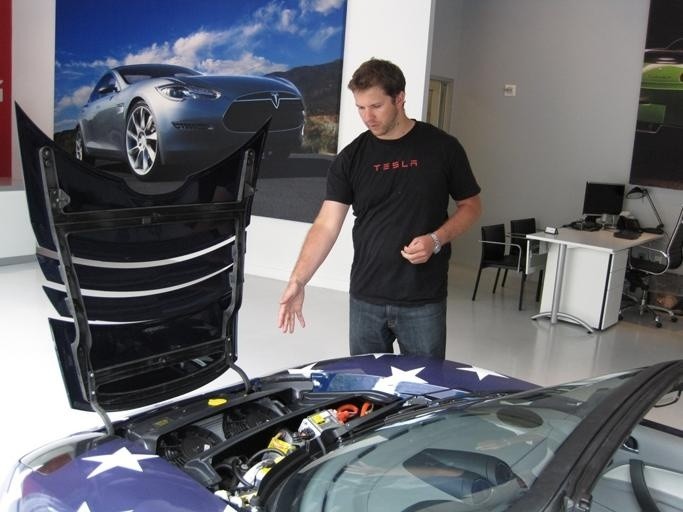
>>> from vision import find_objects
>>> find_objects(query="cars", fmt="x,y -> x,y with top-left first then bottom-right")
73,62 -> 304,182
636,39 -> 682,140
7,99 -> 682,512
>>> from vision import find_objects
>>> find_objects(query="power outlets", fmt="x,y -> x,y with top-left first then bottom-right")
503,84 -> 516,97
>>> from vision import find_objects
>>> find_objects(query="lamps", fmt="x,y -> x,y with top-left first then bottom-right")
625,184 -> 665,235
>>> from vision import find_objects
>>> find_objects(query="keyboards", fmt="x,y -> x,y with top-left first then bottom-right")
614,228 -> 642,240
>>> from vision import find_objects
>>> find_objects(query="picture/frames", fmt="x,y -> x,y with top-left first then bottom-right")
54,0 -> 348,224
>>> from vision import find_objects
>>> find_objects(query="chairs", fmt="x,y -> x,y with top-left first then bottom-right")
617,207 -> 681,329
470,217 -> 548,311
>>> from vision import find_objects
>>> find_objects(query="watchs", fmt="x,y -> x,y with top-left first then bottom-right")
428,233 -> 441,254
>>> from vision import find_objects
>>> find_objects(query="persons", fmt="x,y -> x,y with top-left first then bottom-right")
278,56 -> 483,359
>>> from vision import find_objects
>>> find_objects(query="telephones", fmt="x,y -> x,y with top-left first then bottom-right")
572,221 -> 603,231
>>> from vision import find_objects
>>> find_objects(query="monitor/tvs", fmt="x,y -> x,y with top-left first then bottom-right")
580,180 -> 626,226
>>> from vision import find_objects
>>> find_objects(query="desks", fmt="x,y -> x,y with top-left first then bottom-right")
525,223 -> 667,333
